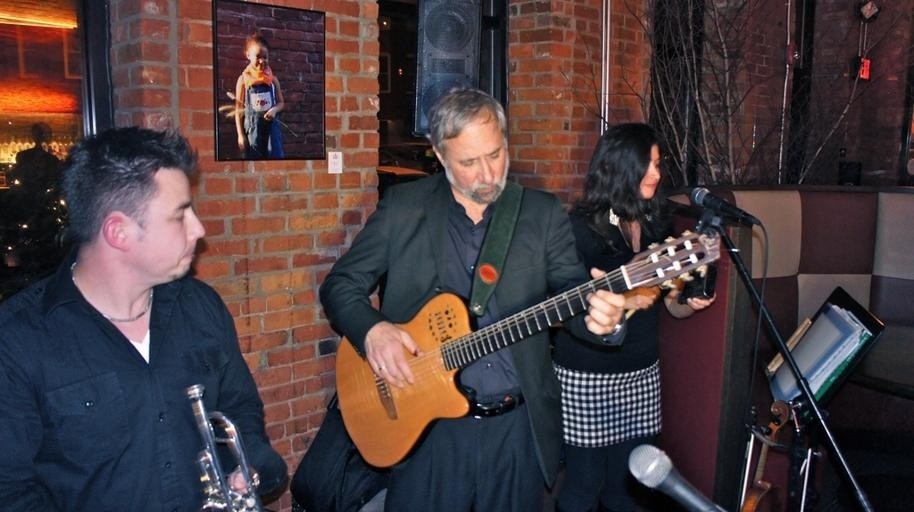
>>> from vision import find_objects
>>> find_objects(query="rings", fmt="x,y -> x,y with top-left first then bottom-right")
378,364 -> 386,373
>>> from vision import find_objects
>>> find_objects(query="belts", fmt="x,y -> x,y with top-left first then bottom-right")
469,393 -> 525,417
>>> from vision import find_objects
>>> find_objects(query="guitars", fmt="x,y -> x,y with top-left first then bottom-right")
335,230 -> 719,467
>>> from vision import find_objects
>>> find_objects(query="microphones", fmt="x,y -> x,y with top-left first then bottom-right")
692,186 -> 760,226
627,444 -> 721,512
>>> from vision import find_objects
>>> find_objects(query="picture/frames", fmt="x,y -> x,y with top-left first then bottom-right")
211,0 -> 327,162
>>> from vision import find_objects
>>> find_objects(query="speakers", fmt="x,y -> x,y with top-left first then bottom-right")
416,0 -> 484,139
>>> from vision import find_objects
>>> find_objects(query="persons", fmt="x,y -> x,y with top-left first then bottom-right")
0,129 -> 289,510
548,121 -> 718,511
234,34 -> 284,160
13,124 -> 62,190
319,88 -> 625,512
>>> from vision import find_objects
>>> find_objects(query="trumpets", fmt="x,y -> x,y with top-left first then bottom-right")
186,384 -> 261,511
